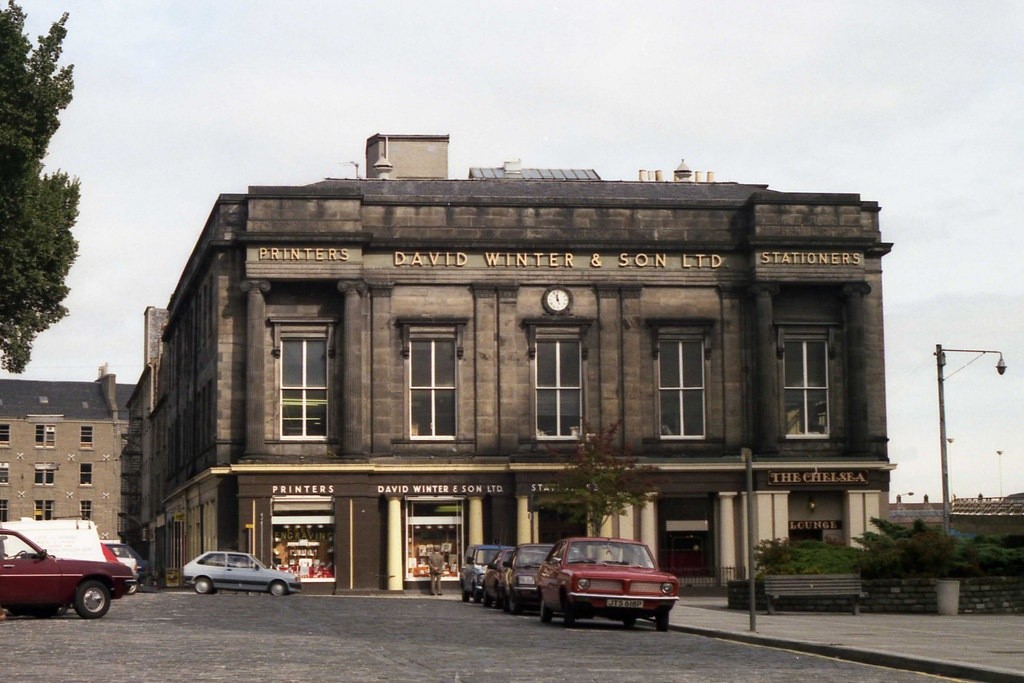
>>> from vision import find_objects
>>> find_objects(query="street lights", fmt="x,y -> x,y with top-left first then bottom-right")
947,438 -> 953,506
898,492 -> 913,520
996,451 -> 1004,497
933,345 -> 1007,537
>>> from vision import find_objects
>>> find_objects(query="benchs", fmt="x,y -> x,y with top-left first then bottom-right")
761,574 -> 869,616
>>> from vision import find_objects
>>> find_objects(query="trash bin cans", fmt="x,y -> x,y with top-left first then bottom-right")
726,579 -> 747,609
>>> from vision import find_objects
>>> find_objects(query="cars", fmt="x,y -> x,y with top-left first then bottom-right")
503,543 -> 554,614
0,529 -> 137,619
536,538 -> 679,632
99,540 -> 139,595
483,548 -> 514,608
460,544 -> 511,604
183,550 -> 302,596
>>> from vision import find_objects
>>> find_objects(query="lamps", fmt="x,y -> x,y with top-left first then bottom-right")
809,500 -> 816,511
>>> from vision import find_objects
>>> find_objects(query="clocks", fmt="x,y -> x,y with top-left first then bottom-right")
542,286 -> 573,316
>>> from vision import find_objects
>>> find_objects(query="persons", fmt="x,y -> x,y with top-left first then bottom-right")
428,544 -> 445,595
